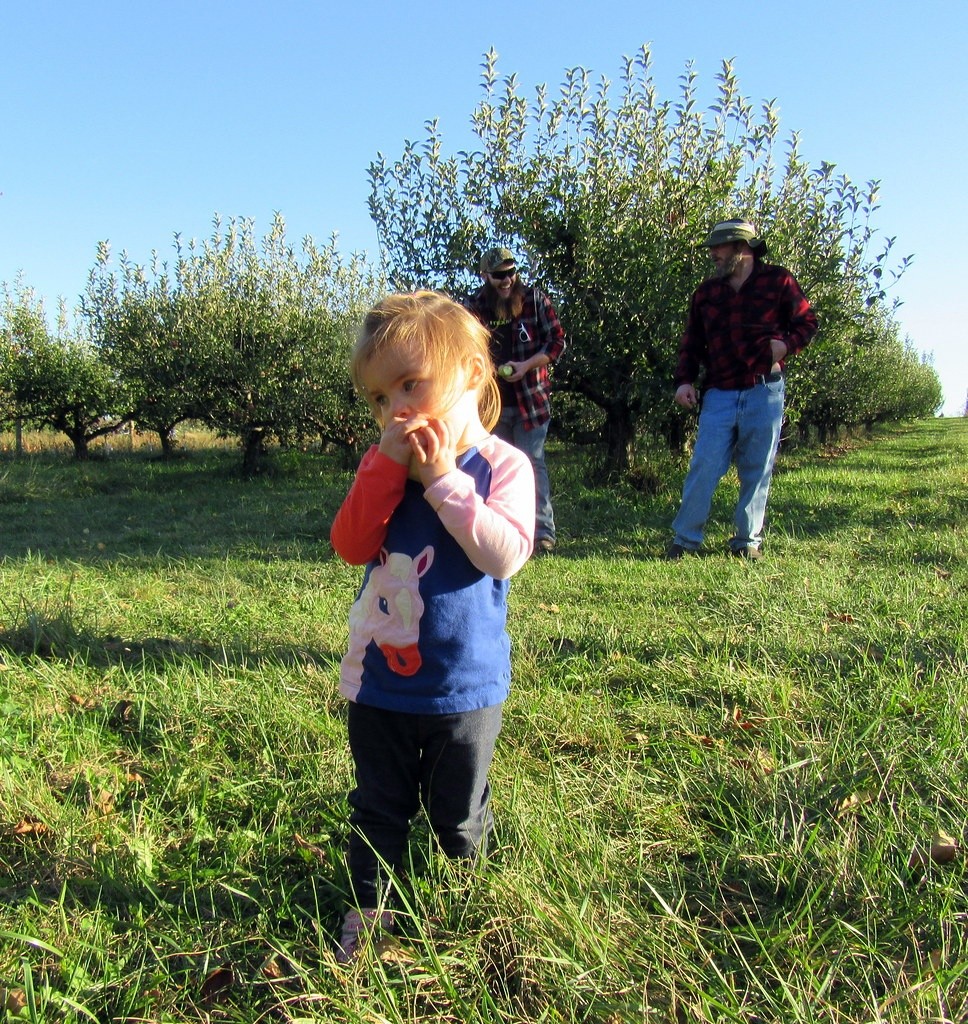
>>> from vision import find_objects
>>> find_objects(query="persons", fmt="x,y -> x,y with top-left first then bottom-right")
665,218 -> 819,562
460,247 -> 566,552
330,290 -> 538,972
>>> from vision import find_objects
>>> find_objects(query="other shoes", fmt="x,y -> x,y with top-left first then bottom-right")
535,539 -> 554,554
737,546 -> 765,563
668,544 -> 687,559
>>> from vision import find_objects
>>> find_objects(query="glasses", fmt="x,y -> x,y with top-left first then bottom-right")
485,267 -> 516,280
512,322 -> 531,343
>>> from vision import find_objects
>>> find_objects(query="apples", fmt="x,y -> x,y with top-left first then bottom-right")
498,365 -> 512,376
407,447 -> 427,482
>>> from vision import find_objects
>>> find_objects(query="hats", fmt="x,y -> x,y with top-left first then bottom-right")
696,217 -> 767,257
479,248 -> 513,271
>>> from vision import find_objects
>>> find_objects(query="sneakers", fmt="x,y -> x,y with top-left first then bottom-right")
336,909 -> 394,964
